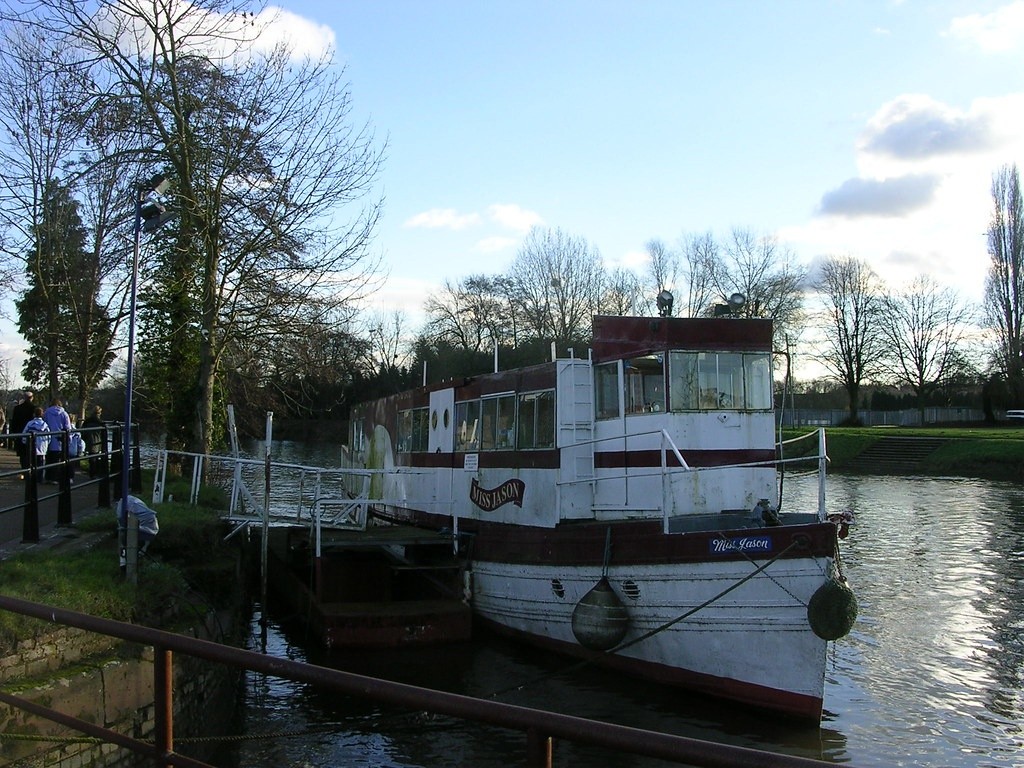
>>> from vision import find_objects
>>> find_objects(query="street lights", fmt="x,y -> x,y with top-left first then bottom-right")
119,173 -> 180,575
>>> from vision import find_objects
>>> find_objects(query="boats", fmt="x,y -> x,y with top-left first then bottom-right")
340,308 -> 860,729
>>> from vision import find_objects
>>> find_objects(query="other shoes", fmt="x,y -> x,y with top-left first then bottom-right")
52,481 -> 59,485
70,478 -> 74,484
16,474 -> 25,480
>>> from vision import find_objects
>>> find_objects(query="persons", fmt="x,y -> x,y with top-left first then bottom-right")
116,494 -> 159,571
345,415 -> 394,499
0,392 -> 105,484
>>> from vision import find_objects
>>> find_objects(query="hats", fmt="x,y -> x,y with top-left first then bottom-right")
93,405 -> 102,413
